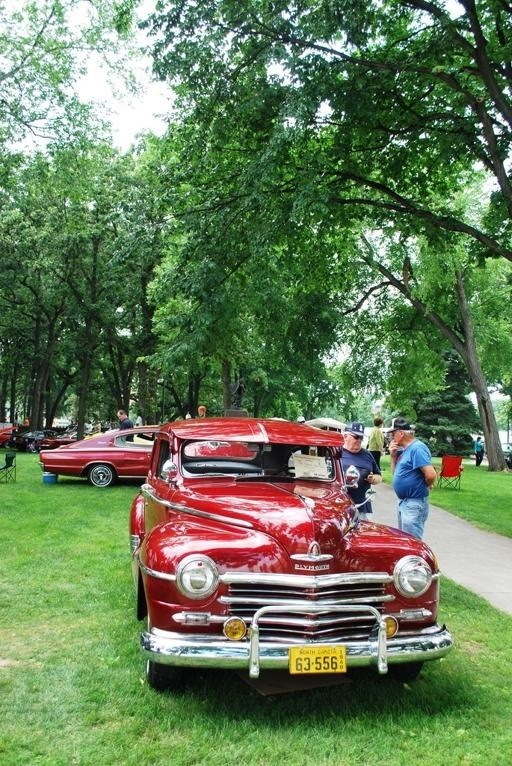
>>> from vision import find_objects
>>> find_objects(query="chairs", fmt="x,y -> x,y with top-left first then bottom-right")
436,452 -> 465,492
0,450 -> 18,484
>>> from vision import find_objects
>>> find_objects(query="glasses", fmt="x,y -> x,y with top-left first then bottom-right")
352,435 -> 362,439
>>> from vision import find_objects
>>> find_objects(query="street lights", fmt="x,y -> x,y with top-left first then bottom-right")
157,379 -> 165,424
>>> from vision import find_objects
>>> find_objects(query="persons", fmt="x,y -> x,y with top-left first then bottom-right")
385,417 -> 437,540
338,422 -> 383,520
365,417 -> 385,470
473,436 -> 484,467
116,408 -> 135,443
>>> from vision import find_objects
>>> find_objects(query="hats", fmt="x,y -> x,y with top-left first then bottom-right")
386,418 -> 411,433
345,422 -> 367,437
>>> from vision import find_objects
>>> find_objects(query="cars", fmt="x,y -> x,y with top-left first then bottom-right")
382,428 -> 395,457
41,425 -> 163,489
129,416 -> 454,691
500,442 -> 511,469
40,432 -> 92,448
9,430 -> 60,453
1,423 -> 23,449
